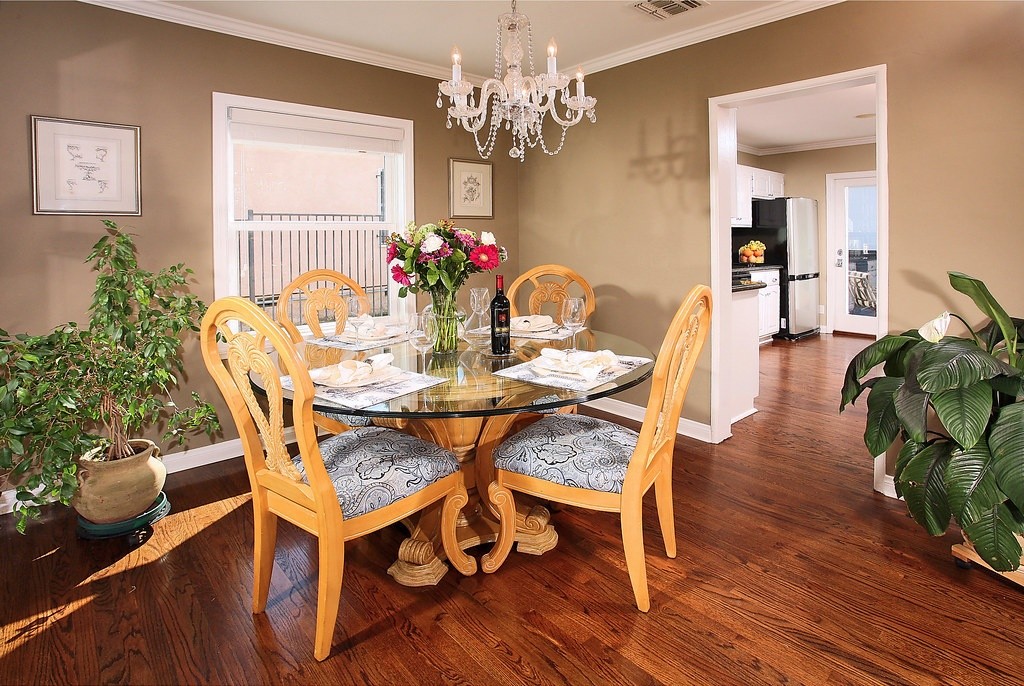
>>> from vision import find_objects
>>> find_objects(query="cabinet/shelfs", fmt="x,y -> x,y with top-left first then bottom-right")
731,163 -> 752,228
752,167 -> 787,200
749,268 -> 780,346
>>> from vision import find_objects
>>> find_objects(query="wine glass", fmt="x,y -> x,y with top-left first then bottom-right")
470,288 -> 491,334
345,295 -> 370,347
408,313 -> 438,379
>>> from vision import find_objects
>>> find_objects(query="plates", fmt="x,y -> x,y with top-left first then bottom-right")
503,323 -> 558,332
345,327 -> 403,341
532,351 -> 618,373
310,362 -> 401,389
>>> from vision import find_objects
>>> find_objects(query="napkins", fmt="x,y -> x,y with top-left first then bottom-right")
542,347 -> 618,370
337,353 -> 393,385
350,318 -> 386,338
516,314 -> 551,330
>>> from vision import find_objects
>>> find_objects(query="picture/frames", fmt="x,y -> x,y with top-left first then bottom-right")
30,114 -> 142,217
448,157 -> 495,220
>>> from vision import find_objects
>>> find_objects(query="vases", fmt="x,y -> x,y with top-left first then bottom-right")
429,290 -> 459,355
951,499 -> 1024,587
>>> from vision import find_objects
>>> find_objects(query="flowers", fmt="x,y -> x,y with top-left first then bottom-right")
838,271 -> 1024,574
375,219 -> 508,353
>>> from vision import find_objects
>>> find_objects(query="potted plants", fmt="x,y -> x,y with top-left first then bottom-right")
0,219 -> 228,540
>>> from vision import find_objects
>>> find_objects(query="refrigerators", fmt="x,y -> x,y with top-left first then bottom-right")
751,196 -> 821,336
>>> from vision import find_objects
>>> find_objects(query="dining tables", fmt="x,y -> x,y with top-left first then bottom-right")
248,319 -> 657,586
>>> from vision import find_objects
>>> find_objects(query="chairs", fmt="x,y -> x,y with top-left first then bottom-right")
847,270 -> 877,317
200,295 -> 478,662
277,269 -> 374,436
482,284 -> 714,612
479,263 -> 597,440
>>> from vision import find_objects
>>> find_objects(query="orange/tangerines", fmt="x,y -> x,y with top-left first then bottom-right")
740,247 -> 765,263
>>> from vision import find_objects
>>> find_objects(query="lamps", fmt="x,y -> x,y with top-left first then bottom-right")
436,0 -> 598,163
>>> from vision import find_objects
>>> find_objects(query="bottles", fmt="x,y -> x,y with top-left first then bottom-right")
490,274 -> 512,355
560,298 -> 586,359
